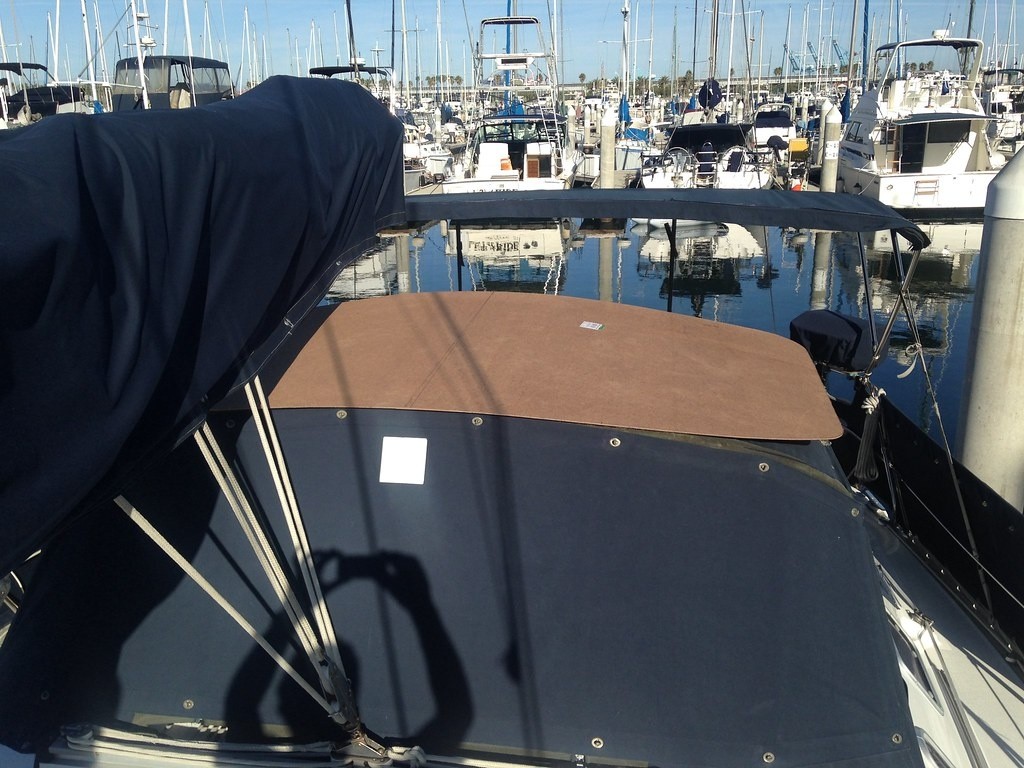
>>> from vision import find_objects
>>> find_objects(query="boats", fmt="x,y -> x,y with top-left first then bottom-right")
857,253 -> 969,320
326,244 -> 397,298
640,225 -> 765,259
441,218 -> 575,272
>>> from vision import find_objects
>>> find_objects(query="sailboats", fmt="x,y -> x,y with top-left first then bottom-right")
0,0 -> 1023,266
